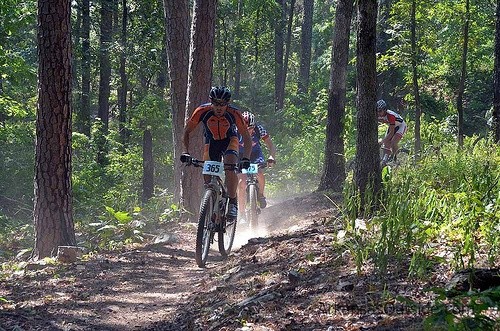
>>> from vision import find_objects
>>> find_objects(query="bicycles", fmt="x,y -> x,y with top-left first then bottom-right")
237,161 -> 262,231
184,157 -> 239,267
349,147 -> 397,171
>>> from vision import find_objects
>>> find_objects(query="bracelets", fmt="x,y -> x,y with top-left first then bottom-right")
242,158 -> 249,161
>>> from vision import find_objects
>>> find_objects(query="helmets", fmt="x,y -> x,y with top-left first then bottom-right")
377,100 -> 386,111
241,111 -> 256,126
209,86 -> 231,100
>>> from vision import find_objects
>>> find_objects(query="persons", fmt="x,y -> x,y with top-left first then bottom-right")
235,111 -> 275,213
179,86 -> 253,245
376,99 -> 407,166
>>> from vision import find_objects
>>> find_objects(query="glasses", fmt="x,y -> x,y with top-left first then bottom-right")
212,102 -> 227,107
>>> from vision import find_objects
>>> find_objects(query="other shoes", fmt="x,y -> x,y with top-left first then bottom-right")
259,196 -> 267,209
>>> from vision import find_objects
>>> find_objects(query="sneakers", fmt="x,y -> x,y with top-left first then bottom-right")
228,204 -> 238,216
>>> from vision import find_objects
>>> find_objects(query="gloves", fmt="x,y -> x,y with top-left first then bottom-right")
236,157 -> 250,173
180,152 -> 194,167
